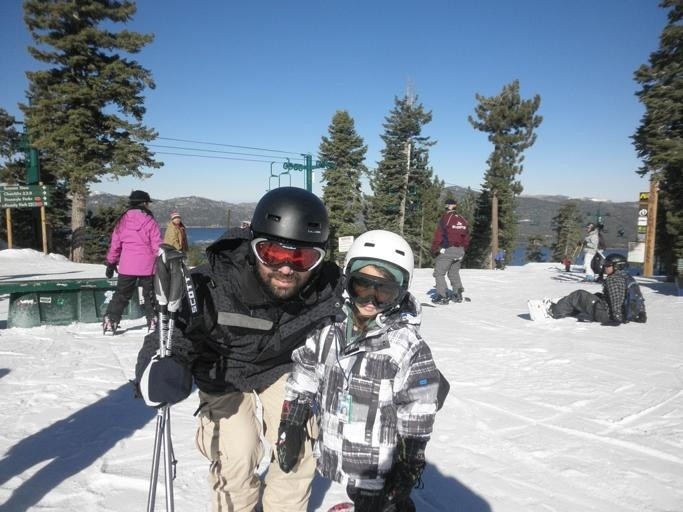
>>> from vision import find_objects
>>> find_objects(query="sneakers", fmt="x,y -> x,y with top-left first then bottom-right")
534,298 -> 551,318
148,316 -> 157,329
451,293 -> 462,303
102,314 -> 117,331
432,295 -> 449,305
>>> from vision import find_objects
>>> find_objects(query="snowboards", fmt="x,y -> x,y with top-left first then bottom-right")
420,297 -> 471,307
529,294 -> 603,321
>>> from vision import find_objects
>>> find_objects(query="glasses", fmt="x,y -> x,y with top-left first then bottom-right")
251,238 -> 325,272
346,272 -> 399,308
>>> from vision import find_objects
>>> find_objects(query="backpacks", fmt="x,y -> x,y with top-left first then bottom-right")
624,275 -> 642,321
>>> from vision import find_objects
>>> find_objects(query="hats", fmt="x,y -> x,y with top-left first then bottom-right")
169,212 -> 180,219
130,190 -> 152,201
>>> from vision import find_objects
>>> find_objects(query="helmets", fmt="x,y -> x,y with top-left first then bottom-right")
445,199 -> 455,211
606,253 -> 626,270
587,223 -> 594,232
251,187 -> 330,247
343,229 -> 414,289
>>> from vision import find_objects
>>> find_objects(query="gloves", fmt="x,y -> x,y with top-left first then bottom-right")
638,311 -> 647,323
275,400 -> 309,473
378,463 -> 414,512
105,262 -> 117,278
148,359 -> 192,403
601,319 -> 621,327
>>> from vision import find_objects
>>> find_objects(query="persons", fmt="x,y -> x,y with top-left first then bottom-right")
104,191 -> 163,336
592,221 -> 606,280
578,223 -> 599,282
164,213 -> 188,255
528,255 -> 646,326
431,198 -> 468,305
135,188 -> 344,511
278,228 -> 439,510
